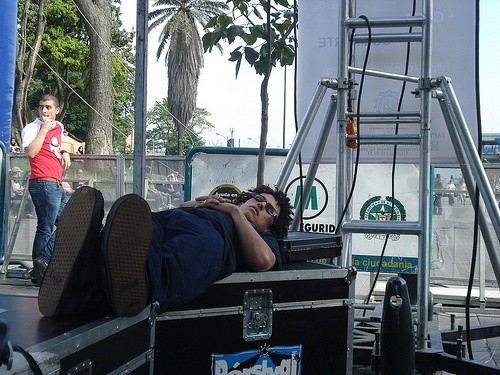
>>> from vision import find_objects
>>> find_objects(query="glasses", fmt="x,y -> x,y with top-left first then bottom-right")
254,193 -> 278,223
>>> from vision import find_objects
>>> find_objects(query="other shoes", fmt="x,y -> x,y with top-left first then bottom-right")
100,194 -> 153,318
30,257 -> 47,287
37,186 -> 104,319
25,213 -> 37,219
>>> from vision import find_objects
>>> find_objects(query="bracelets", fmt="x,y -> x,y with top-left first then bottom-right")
60,150 -> 67,154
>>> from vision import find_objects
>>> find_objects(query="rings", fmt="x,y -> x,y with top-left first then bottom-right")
204,200 -> 207,204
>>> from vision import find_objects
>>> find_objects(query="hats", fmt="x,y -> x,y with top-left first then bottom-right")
12,167 -> 24,173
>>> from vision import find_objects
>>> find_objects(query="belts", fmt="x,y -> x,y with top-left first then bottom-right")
36,177 -> 60,185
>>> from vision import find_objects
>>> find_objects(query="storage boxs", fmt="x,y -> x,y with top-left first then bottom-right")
0,261 -> 358,375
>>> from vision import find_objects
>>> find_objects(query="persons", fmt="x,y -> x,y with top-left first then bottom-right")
9,137 -> 468,215
36,185 -> 294,320
21,94 -> 71,286
